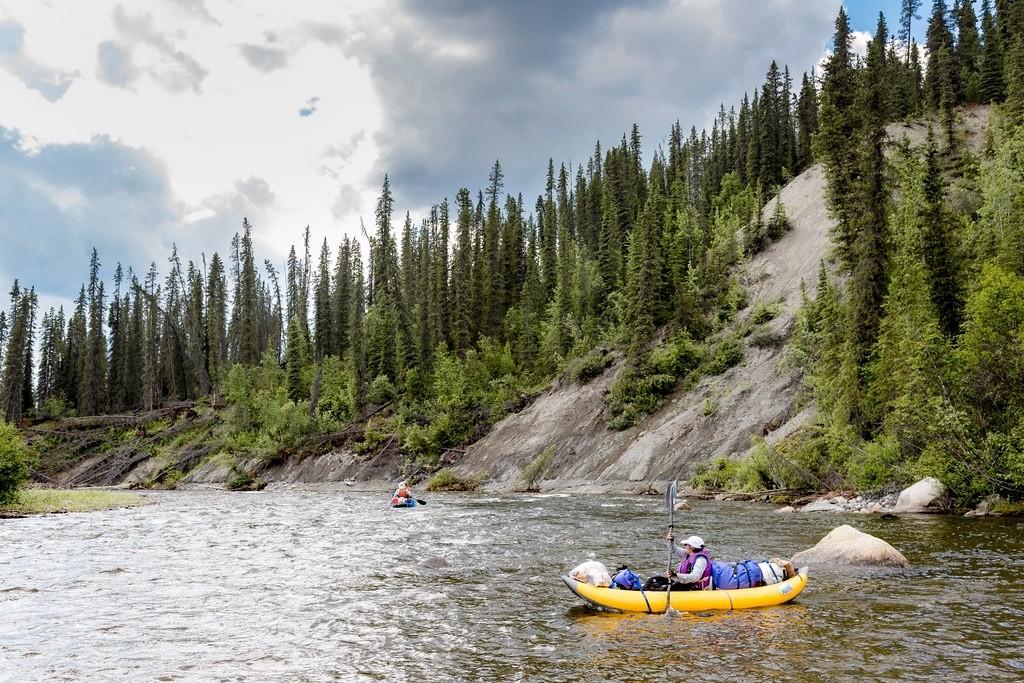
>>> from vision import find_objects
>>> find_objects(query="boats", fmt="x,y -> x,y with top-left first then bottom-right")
390,493 -> 415,507
561,567 -> 812,613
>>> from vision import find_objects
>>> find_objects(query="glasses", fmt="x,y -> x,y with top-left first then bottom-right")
684,544 -> 692,548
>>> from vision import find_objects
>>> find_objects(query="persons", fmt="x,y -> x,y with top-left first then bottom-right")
661,532 -> 711,590
396,482 -> 412,499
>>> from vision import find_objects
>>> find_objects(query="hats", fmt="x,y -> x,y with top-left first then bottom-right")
399,482 -> 405,489
680,535 -> 705,549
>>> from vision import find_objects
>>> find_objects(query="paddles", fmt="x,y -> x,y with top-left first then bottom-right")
665,478 -> 679,615
412,496 -> 429,507
